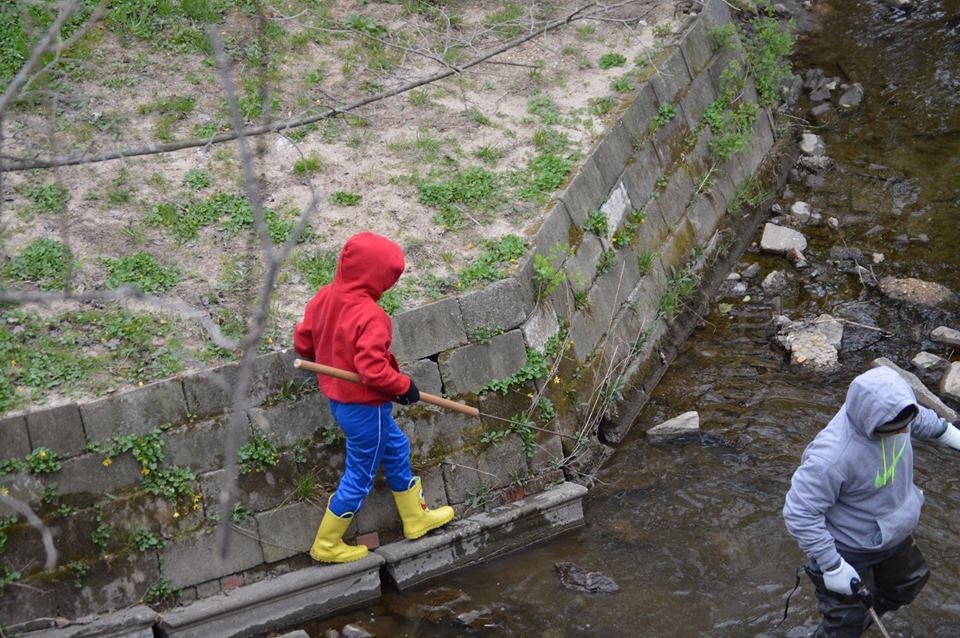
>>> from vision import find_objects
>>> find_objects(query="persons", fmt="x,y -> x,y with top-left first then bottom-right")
781,366 -> 960,638
293,231 -> 455,563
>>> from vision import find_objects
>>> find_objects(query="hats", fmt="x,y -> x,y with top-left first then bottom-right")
876,416 -> 916,434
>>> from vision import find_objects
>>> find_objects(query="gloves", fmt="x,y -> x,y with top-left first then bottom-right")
396,379 -> 420,404
822,557 -> 869,596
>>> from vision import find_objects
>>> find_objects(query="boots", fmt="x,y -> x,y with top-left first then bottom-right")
391,473 -> 454,538
309,492 -> 369,562
